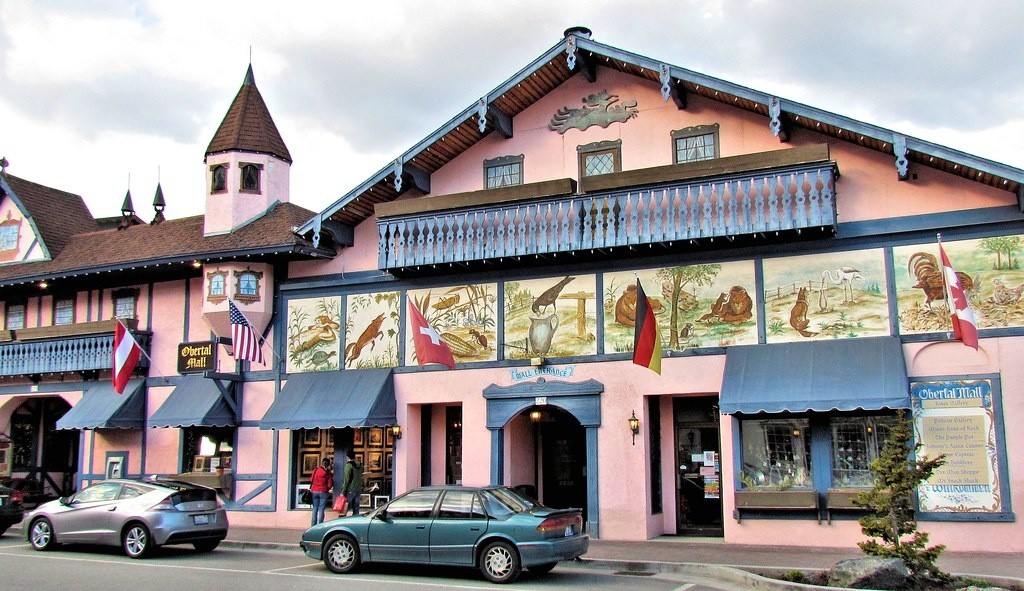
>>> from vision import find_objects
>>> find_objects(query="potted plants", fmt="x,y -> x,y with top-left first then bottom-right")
216,466 -> 223,476
827,470 -> 890,507
736,464 -> 817,508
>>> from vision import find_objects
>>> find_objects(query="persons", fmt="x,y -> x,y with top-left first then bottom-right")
310,458 -> 334,526
339,451 -> 362,518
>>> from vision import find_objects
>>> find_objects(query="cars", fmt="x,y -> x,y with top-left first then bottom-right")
299,480 -> 590,583
20,475 -> 229,559
0,483 -> 25,536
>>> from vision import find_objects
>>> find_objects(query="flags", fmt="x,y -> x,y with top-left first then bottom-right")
632,279 -> 662,376
227,301 -> 267,367
111,320 -> 140,394
939,243 -> 978,351
407,299 -> 456,369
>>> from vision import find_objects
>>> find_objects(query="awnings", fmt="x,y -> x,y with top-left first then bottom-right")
145,375 -> 236,429
54,379 -> 144,431
718,333 -> 913,415
259,367 -> 395,432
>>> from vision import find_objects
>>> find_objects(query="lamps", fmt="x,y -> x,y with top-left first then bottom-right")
792,427 -> 800,439
530,409 -> 542,427
392,420 -> 401,440
866,425 -> 874,442
627,410 -> 639,445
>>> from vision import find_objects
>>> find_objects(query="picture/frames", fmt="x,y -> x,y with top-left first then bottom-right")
385,450 -> 393,475
385,477 -> 392,499
367,427 -> 384,448
359,493 -> 371,507
354,427 -> 365,448
293,481 -> 314,509
385,426 -> 393,448
366,476 -> 384,495
325,429 -> 335,447
367,451 -> 383,472
375,495 -> 389,510
325,451 -> 335,476
193,456 -> 205,472
300,451 -> 321,477
354,451 -> 365,473
301,427 -> 322,448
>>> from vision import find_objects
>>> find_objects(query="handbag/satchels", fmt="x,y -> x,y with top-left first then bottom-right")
335,501 -> 348,516
332,495 -> 347,511
302,490 -> 313,505
